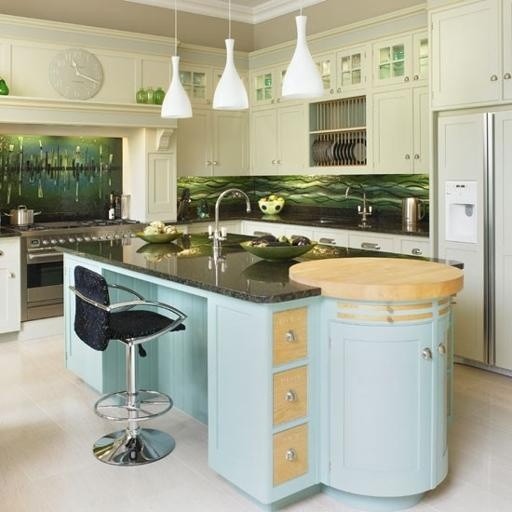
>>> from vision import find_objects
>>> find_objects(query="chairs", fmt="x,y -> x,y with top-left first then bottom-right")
68,265 -> 188,467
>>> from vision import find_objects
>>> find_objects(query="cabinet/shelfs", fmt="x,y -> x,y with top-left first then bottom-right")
177,27 -> 429,176
428,0 -> 512,112
0,236 -> 23,334
172,217 -> 432,258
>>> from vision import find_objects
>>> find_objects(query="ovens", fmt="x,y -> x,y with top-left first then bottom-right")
22,248 -> 64,322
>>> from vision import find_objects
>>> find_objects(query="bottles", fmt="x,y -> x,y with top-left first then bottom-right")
0,79 -> 9,95
107,193 -> 116,220
136,87 -> 165,105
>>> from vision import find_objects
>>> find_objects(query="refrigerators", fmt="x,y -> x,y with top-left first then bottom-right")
436,107 -> 511,376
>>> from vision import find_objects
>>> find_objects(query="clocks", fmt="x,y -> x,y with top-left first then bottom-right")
48,48 -> 104,100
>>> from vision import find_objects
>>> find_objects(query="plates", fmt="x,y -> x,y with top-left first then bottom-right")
311,139 -> 366,162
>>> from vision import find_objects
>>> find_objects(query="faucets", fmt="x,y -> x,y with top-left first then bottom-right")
345,183 -> 373,224
209,248 -> 227,290
208,187 -> 252,245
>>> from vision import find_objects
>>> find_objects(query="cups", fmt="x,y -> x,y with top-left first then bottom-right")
391,50 -> 404,61
265,79 -> 271,87
400,196 -> 425,234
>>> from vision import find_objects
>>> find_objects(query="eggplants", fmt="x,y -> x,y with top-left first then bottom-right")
251,234 -> 312,247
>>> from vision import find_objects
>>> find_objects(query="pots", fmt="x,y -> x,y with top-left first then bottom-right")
3,204 -> 42,226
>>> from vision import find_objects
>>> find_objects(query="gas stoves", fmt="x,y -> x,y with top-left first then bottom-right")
1,219 -> 149,248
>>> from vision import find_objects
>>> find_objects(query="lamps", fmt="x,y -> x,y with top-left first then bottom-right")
161,0 -> 326,121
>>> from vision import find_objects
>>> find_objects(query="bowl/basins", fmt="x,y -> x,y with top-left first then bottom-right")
258,200 -> 285,215
240,258 -> 302,283
136,231 -> 184,244
239,240 -> 318,262
136,242 -> 183,254
